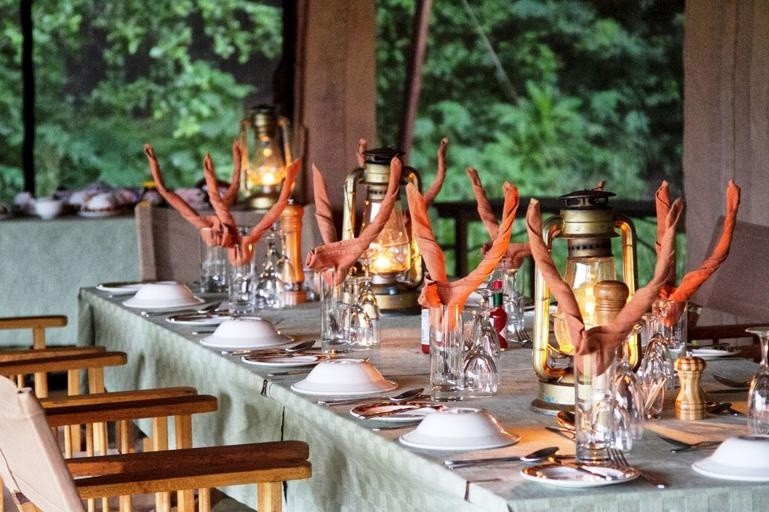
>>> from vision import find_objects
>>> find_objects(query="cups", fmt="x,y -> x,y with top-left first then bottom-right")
33,201 -> 60,219
198,225 -> 689,463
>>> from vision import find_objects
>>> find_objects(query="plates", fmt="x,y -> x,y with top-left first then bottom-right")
96,281 -> 769,489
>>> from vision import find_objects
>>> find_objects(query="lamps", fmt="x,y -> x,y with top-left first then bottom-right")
242,106 -> 291,211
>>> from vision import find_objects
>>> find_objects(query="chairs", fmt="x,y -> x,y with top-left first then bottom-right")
675,214 -> 768,344
0,376 -> 310,509
136,200 -> 326,286
0,349 -> 198,461
0,315 -> 106,370
